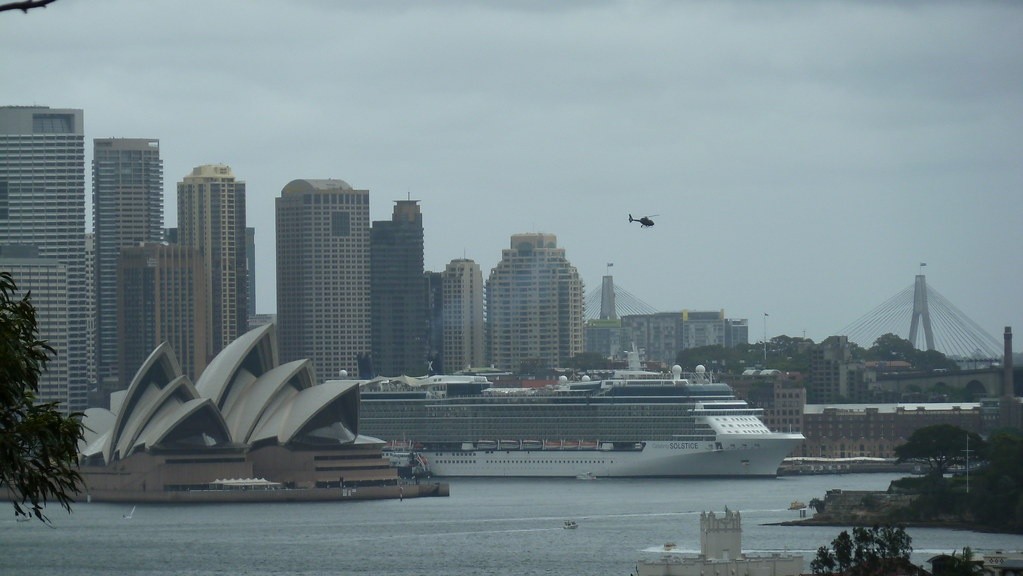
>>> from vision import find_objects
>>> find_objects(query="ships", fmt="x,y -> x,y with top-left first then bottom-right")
324,364 -> 807,480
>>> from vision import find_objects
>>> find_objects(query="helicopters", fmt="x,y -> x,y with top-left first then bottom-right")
628,214 -> 660,228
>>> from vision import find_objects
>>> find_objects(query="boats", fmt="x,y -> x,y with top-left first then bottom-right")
576,472 -> 597,480
563,519 -> 579,530
382,442 -> 392,451
563,441 -> 579,452
546,441 -> 562,450
579,442 -> 598,451
499,440 -> 519,451
394,440 -> 410,450
477,440 -> 498,451
522,440 -> 543,451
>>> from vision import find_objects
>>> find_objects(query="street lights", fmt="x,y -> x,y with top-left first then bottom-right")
763,312 -> 769,360
